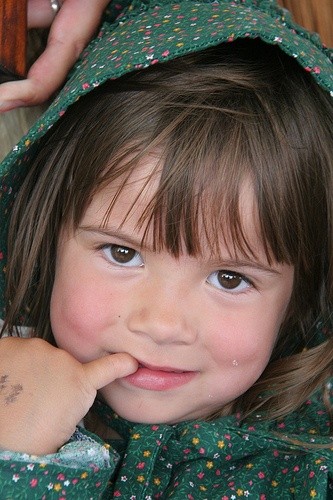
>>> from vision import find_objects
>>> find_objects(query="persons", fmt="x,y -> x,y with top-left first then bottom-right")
0,0 -> 333,499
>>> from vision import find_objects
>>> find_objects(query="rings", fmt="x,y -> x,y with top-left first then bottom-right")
49,0 -> 59,15
